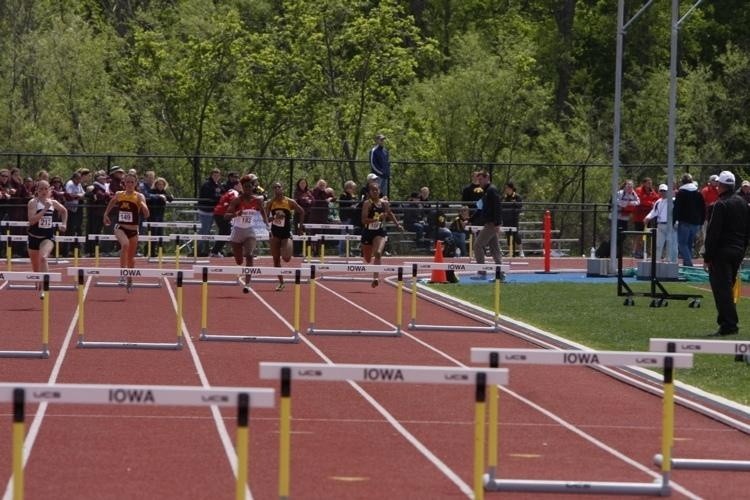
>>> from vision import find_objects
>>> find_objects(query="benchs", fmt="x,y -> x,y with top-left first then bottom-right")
551,229 -> 580,255
517,213 -> 544,254
386,213 -> 418,255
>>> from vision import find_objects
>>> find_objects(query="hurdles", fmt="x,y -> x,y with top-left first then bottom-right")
1,271 -> 62,360
192,265 -> 313,345
470,346 -> 693,497
1,219 -> 366,289
67,266 -> 194,351
259,360 -> 511,500
0,381 -> 277,500
300,262 -> 413,337
649,337 -> 750,472
464,226 -> 529,265
404,261 -> 510,333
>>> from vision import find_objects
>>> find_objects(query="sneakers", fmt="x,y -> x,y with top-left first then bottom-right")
708,327 -> 739,337
243,282 -> 252,293
276,283 -> 285,290
371,278 -> 380,288
119,277 -> 133,293
57,252 -> 225,258
471,274 -> 504,282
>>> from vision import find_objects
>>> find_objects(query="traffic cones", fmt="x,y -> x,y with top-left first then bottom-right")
428,239 -> 450,284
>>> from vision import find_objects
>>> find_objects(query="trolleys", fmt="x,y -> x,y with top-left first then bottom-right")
617,226 -> 704,309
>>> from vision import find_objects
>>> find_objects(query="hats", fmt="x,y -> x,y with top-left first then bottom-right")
742,180 -> 750,187
658,183 -> 668,191
109,165 -> 124,175
715,170 -> 735,185
709,174 -> 719,182
